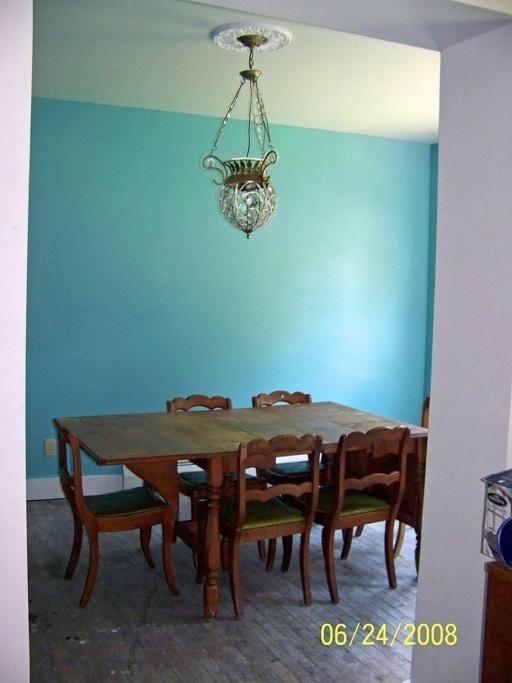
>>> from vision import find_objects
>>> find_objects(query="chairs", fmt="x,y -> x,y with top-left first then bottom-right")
167,390 -> 268,566
198,432 -> 323,619
253,390 -> 329,560
282,426 -> 411,604
356,397 -> 419,575
54,418 -> 178,607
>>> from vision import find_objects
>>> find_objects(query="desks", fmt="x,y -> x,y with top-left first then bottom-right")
54,401 -> 427,621
55,402 -> 428,583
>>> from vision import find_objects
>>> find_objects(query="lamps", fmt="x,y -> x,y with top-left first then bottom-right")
201,23 -> 293,238
